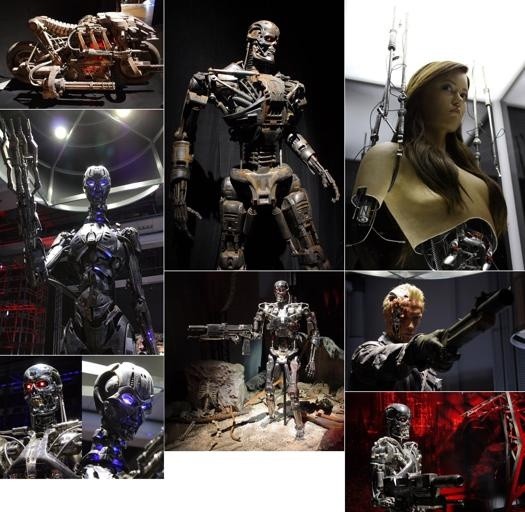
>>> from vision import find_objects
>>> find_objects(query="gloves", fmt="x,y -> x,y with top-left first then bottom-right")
410,328 -> 461,373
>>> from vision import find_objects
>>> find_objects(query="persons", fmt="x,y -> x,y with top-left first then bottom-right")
174,18 -> 339,269
370,401 -> 425,510
81,361 -> 164,478
352,283 -> 461,392
185,280 -> 320,440
351,60 -> 508,268
1,117 -> 159,355
0,362 -> 82,478
